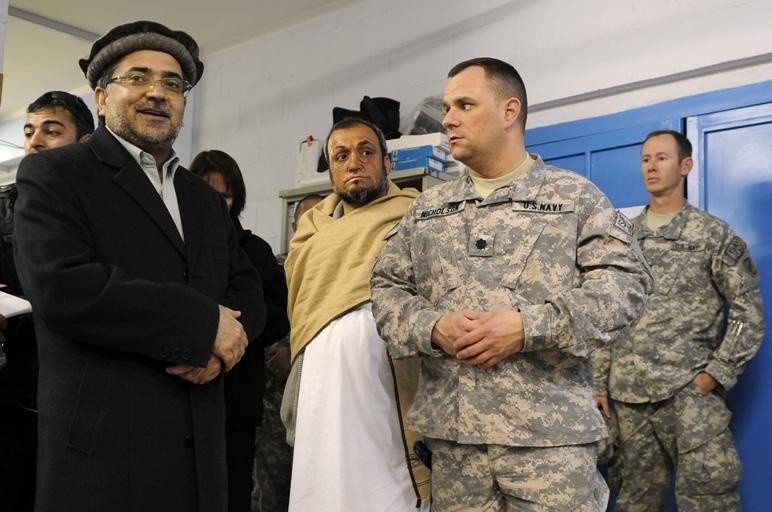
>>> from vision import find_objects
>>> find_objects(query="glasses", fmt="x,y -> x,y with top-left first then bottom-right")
106,71 -> 192,94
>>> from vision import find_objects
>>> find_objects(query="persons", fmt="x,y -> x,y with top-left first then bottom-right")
16,20 -> 268,512
588,130 -> 764,512
281,117 -> 431,512
189,149 -> 290,512
370,57 -> 656,512
0,92 -> 97,512
252,192 -> 328,511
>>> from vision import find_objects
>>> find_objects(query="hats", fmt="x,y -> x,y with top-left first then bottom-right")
78,21 -> 205,91
